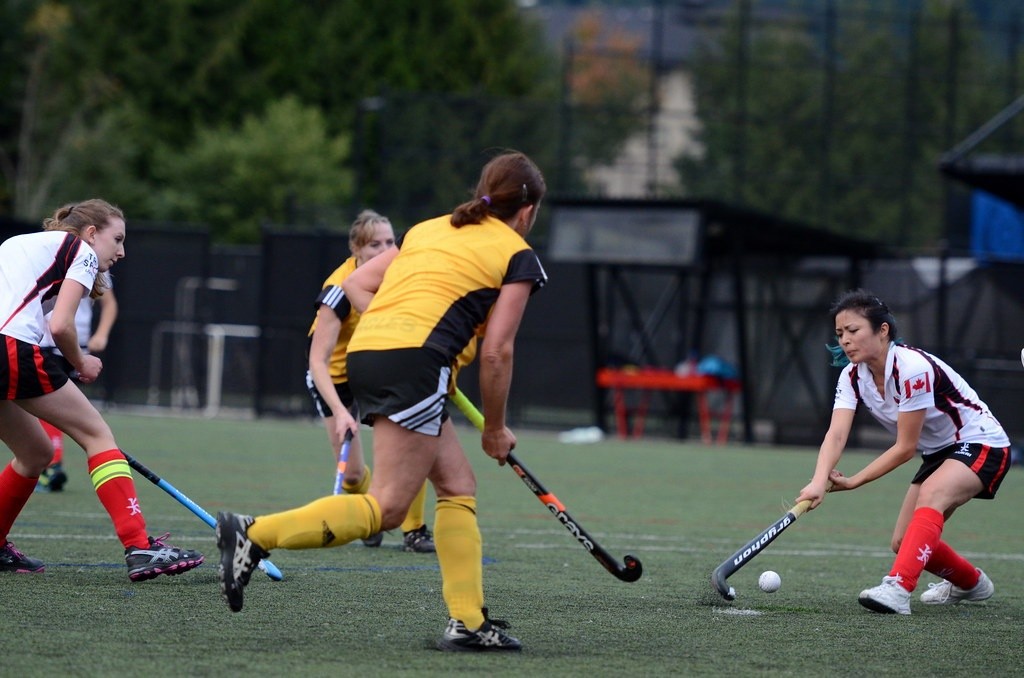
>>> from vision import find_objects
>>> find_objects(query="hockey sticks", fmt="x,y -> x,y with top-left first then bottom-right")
711,471 -> 844,601
449,384 -> 643,582
332,398 -> 359,494
119,448 -> 283,582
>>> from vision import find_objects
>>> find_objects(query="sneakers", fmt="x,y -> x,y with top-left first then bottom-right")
215,510 -> 271,613
857,573 -> 913,616
123,532 -> 205,583
403,524 -> 437,553
0,541 -> 46,573
919,567 -> 995,605
435,606 -> 523,652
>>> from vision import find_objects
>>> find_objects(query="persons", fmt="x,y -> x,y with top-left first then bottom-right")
0,198 -> 205,581
794,293 -> 1011,614
36,271 -> 118,492
305,209 -> 436,554
218,150 -> 548,652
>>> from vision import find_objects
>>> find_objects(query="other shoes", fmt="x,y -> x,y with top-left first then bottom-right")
47,462 -> 69,494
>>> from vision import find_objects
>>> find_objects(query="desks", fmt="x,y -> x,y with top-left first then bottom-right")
596,367 -> 740,447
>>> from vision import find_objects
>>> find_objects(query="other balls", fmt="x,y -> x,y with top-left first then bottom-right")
759,571 -> 781,594
